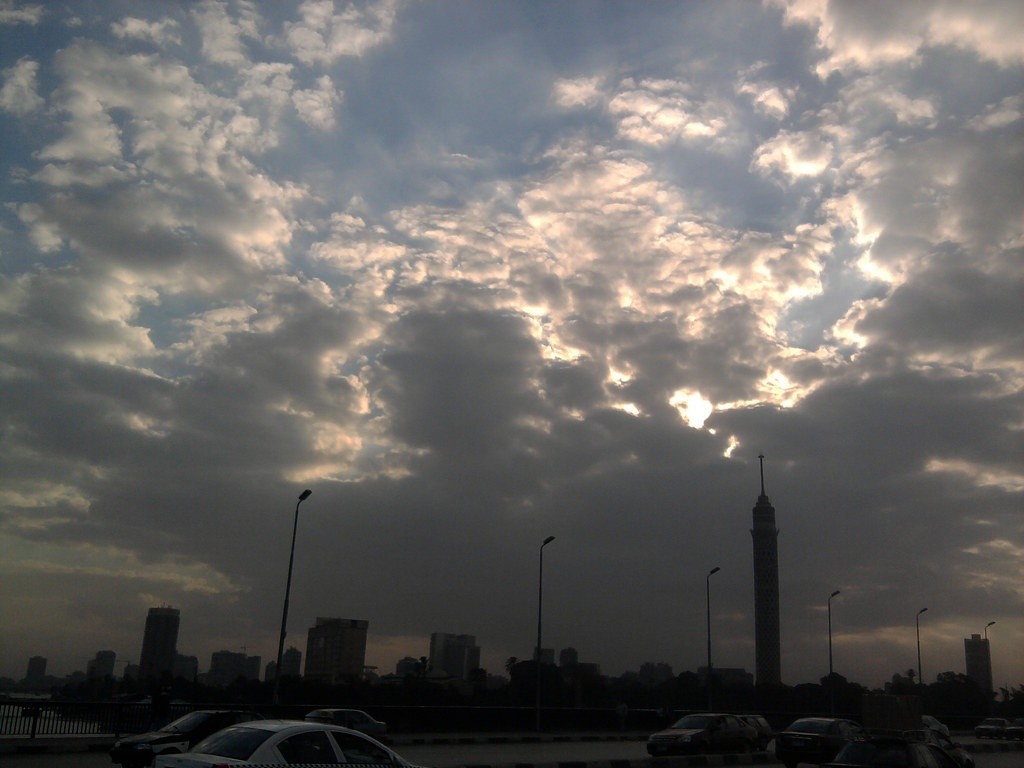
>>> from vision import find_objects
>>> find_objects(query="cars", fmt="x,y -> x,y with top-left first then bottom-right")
920,715 -> 950,737
820,736 -> 962,768
150,719 -> 422,768
735,715 -> 773,751
647,713 -> 758,756
975,718 -> 1010,739
305,709 -> 386,738
775,717 -> 872,768
107,710 -> 266,768
848,729 -> 976,768
1006,718 -> 1024,741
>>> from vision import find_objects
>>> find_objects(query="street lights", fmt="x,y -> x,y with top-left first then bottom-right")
535,535 -> 555,731
272,489 -> 312,704
916,608 -> 928,687
985,622 -> 995,639
828,591 -> 840,713
707,567 -> 720,712
